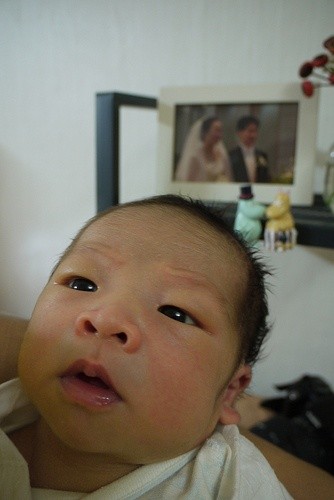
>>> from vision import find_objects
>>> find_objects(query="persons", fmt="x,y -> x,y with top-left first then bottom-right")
0,194 -> 299,500
228,115 -> 273,182
1,311 -> 333,500
175,115 -> 237,184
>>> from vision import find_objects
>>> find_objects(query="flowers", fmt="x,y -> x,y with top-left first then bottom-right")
299,37 -> 334,100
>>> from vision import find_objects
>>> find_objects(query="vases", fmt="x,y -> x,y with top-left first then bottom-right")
323,145 -> 334,215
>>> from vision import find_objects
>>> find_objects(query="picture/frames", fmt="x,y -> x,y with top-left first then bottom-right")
156,82 -> 319,207
